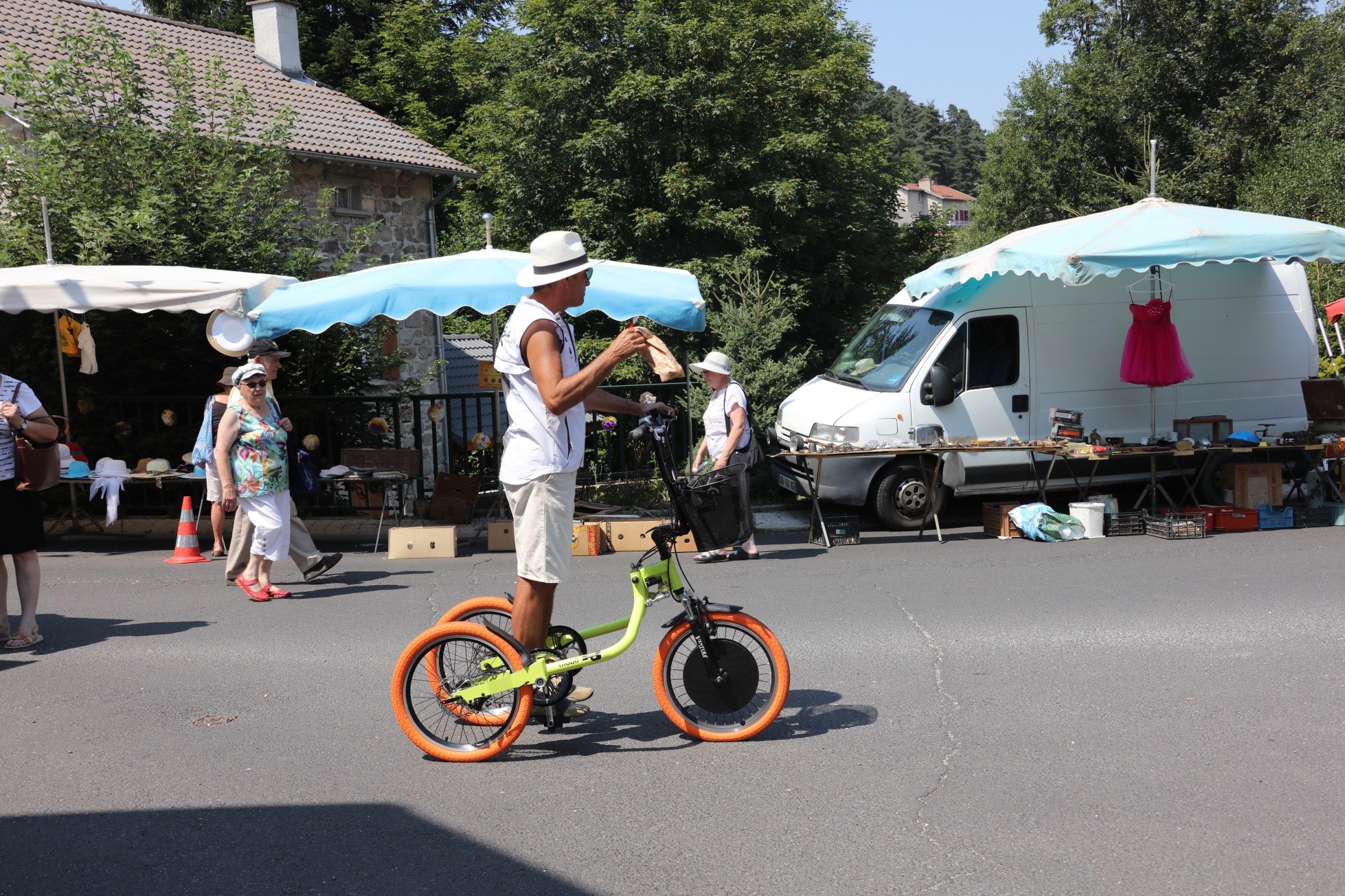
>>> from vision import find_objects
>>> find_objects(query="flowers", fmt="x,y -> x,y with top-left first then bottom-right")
369,417 -> 388,436
162,409 -> 176,426
427,403 -> 443,422
303,434 -> 322,451
77,399 -> 95,414
115,422 -> 134,438
471,433 -> 491,450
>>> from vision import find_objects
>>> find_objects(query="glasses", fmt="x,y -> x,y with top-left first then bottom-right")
583,268 -> 593,279
238,379 -> 268,388
700,370 -> 711,376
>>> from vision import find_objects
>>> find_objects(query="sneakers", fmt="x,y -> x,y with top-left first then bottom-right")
225,577 -> 240,587
532,672 -> 594,701
303,552 -> 342,581
530,695 -> 590,717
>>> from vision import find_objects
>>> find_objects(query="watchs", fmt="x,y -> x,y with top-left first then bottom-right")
17,418 -> 28,431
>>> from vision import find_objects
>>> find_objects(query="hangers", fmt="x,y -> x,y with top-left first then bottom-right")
1125,267 -> 1175,293
63,310 -> 89,329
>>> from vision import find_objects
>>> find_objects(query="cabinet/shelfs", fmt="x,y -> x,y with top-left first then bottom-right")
340,448 -> 420,516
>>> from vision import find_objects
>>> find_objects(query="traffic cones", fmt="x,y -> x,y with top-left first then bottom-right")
162,495 -> 213,565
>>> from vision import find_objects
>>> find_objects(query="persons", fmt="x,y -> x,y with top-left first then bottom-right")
494,231 -> 686,717
193,338 -> 343,601
0,372 -> 58,649
687,351 -> 759,562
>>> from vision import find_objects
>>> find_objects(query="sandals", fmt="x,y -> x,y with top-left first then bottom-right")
4,624 -> 43,648
0,623 -> 11,641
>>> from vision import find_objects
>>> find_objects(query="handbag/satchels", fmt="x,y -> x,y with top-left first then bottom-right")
701,472 -> 745,540
8,382 -> 70,491
729,428 -> 764,471
192,395 -> 215,470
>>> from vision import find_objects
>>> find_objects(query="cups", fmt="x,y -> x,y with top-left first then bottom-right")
1140,431 -> 1195,448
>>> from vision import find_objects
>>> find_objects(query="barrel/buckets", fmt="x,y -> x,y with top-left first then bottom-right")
1068,501 -> 1105,538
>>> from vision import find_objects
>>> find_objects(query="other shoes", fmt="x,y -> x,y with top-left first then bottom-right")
262,584 -> 292,598
235,573 -> 269,601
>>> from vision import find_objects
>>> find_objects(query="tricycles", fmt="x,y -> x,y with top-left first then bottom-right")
385,404 -> 791,768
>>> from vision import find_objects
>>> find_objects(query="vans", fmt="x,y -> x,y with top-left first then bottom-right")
768,252 -> 1322,532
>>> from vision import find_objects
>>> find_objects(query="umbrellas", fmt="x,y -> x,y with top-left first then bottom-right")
248,213 -> 707,490
903,139 -> 1345,511
0,197 -> 303,504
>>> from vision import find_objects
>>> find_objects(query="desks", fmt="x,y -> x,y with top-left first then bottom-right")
766,444 -> 1345,548
43,477 -> 229,555
289,478 -> 422,556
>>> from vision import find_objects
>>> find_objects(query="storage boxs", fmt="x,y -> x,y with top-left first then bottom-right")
487,520 -> 516,551
431,471 -> 482,525
388,526 -> 457,559
572,515 -> 698,557
808,513 -> 859,545
984,407 -> 1345,541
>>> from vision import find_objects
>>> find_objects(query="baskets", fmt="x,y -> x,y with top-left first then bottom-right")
678,462 -> 755,552
983,498 -> 1344,540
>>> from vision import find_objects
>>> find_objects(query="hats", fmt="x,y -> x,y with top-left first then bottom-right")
247,339 -> 292,358
55,441 -> 210,527
207,309 -> 254,357
232,363 -> 267,385
688,351 -> 731,375
516,231 -> 606,287
217,367 -> 237,386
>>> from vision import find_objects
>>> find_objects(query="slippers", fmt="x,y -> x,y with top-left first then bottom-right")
694,548 -> 730,561
212,549 -> 223,556
729,546 -> 760,559
